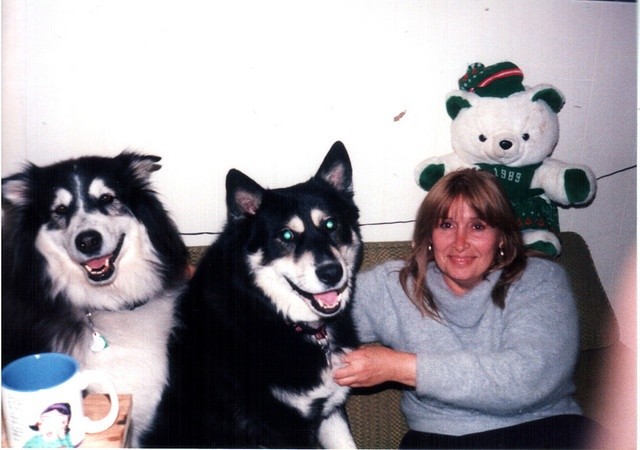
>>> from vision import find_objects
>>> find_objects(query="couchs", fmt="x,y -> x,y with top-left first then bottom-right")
185,233 -> 636,447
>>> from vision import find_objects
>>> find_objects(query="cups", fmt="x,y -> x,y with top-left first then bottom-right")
1,352 -> 120,448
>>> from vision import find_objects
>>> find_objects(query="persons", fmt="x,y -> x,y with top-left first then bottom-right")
334,167 -> 617,449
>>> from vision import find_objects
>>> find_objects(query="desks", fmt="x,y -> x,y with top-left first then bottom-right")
1,392 -> 132,448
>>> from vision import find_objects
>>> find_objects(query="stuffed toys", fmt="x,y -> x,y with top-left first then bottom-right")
414,62 -> 596,255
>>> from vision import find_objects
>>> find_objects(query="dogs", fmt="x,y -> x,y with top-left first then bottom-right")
1,149 -> 192,449
137,142 -> 365,449
